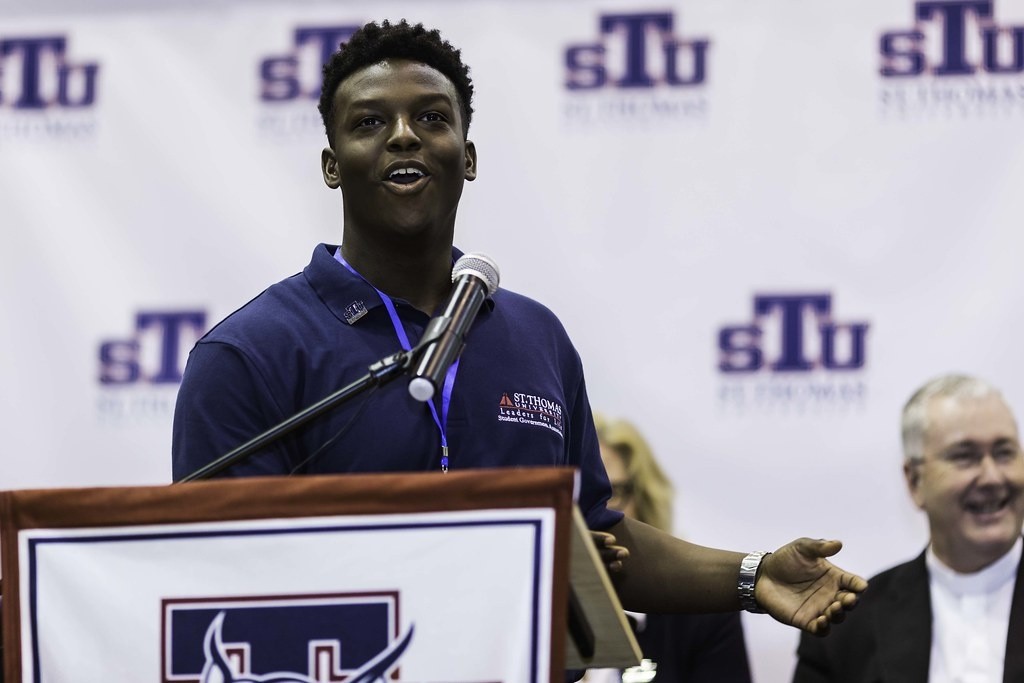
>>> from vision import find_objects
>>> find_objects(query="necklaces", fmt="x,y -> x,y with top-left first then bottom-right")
333,245 -> 458,473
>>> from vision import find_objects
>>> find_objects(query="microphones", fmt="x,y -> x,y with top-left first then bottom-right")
407,252 -> 501,401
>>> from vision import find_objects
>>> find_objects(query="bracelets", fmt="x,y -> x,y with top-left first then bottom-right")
737,550 -> 773,614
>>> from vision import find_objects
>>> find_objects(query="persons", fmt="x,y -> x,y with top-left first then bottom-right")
172,19 -> 869,683
791,375 -> 1024,683
592,413 -> 753,683
